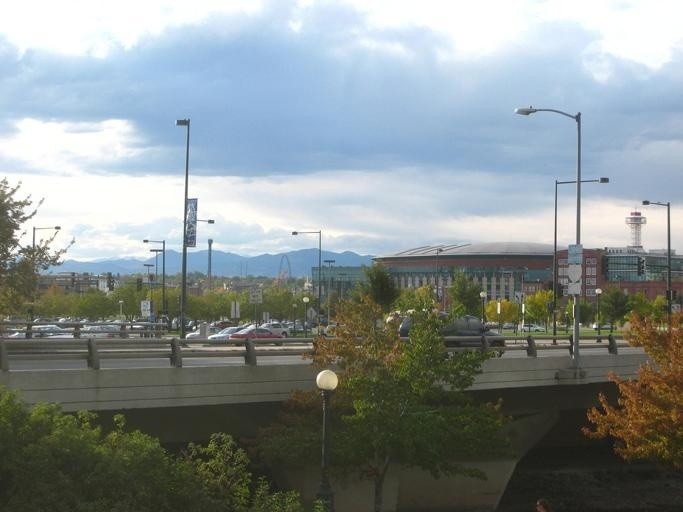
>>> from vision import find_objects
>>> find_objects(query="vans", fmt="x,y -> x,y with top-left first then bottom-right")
400,312 -> 506,357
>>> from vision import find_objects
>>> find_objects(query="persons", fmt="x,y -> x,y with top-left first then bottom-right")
536,498 -> 552,512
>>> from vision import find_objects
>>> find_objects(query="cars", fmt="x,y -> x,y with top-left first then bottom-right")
186,321 -> 290,345
7,315 -> 150,339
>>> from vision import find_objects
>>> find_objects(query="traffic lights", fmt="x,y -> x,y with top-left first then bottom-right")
600,255 -> 608,274
638,257 -> 645,275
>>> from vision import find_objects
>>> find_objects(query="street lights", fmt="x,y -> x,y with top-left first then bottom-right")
292,230 -> 321,337
32,225 -> 61,246
642,200 -> 673,332
144,239 -> 165,312
180,219 -> 215,337
552,177 -> 609,343
316,370 -> 339,508
514,107 -> 581,354
479,292 -> 487,322
